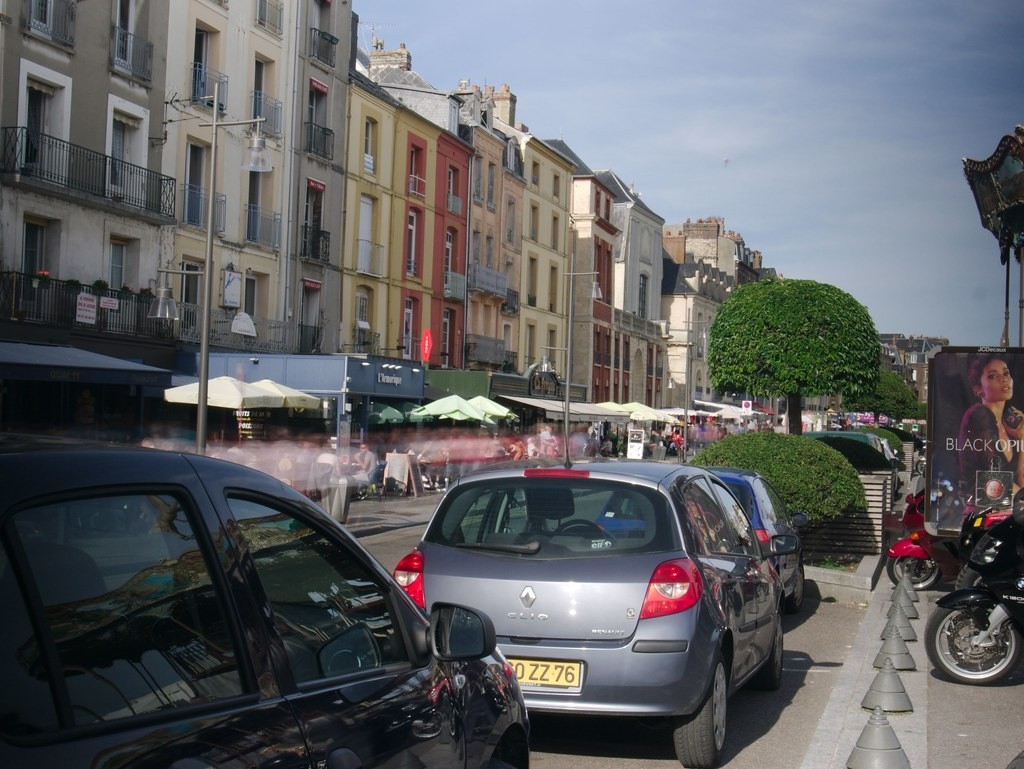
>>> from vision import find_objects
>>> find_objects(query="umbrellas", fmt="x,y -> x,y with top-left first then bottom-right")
395,401 -> 434,423
408,394 -> 496,428
368,403 -> 404,425
468,395 -> 520,426
594,402 -> 742,438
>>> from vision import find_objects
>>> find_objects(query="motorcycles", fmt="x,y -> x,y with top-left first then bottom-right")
883,486 -> 1024,684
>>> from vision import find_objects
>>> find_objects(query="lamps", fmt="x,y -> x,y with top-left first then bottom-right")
431,352 -> 449,357
380,345 -> 406,350
344,340 -> 371,346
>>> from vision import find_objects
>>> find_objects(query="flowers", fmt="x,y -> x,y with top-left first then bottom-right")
118,280 -> 136,300
39,270 -> 51,289
137,287 -> 156,305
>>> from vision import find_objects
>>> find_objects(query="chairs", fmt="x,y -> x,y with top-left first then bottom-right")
628,496 -> 695,553
533,487 -> 591,533
3,535 -> 107,611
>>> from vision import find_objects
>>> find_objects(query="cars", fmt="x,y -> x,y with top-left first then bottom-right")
702,465 -> 810,615
2,445 -> 534,768
393,459 -> 787,768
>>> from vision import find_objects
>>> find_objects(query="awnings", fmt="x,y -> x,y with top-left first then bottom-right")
499,394 -> 631,424
694,398 -> 774,416
1,342 -> 172,387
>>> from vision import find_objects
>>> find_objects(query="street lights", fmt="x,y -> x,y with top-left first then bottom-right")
562,269 -> 605,467
190,116 -> 275,448
681,308 -> 712,463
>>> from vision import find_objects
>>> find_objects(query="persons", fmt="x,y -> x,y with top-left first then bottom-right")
140,426 -> 561,525
957,354 -> 1023,502
570,418 -> 775,460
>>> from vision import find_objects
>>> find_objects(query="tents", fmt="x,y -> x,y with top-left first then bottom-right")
248,378 -> 321,435
163,376 -> 283,447
